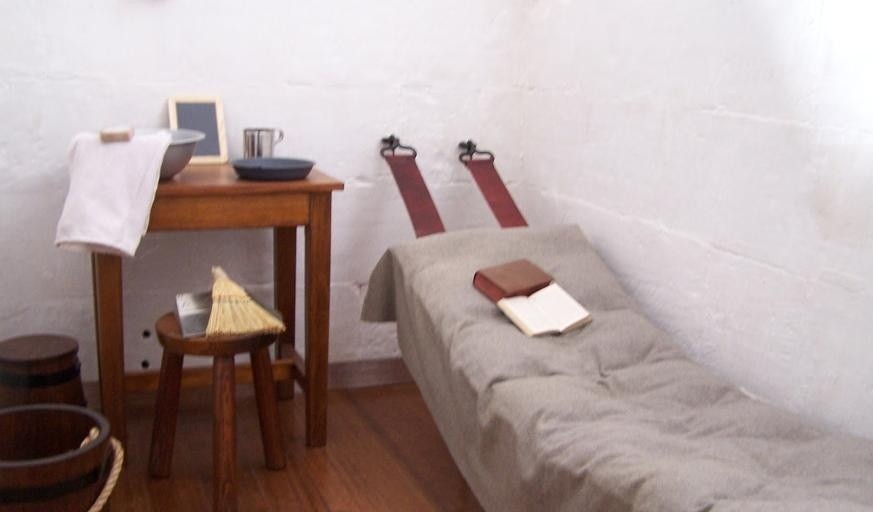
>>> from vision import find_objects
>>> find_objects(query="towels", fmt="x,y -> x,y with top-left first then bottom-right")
54,128 -> 175,261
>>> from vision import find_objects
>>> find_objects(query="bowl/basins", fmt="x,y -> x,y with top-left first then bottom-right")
159,129 -> 206,181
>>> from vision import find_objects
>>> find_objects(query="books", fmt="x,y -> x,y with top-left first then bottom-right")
471,257 -> 554,301
496,282 -> 592,339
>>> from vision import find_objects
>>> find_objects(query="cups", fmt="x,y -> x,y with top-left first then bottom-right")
243,127 -> 283,157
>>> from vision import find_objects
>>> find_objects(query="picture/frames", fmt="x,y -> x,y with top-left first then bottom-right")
164,91 -> 228,167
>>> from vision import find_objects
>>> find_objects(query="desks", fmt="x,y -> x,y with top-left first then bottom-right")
65,156 -> 344,468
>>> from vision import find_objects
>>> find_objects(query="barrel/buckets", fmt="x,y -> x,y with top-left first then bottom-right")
0,406 -> 124,512
0,335 -> 88,409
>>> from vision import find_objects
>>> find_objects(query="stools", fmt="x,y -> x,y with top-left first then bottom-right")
147,308 -> 288,512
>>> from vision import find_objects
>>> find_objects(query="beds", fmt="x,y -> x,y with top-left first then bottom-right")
359,133 -> 873,512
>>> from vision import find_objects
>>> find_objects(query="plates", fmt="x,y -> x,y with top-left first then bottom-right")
232,157 -> 316,181
